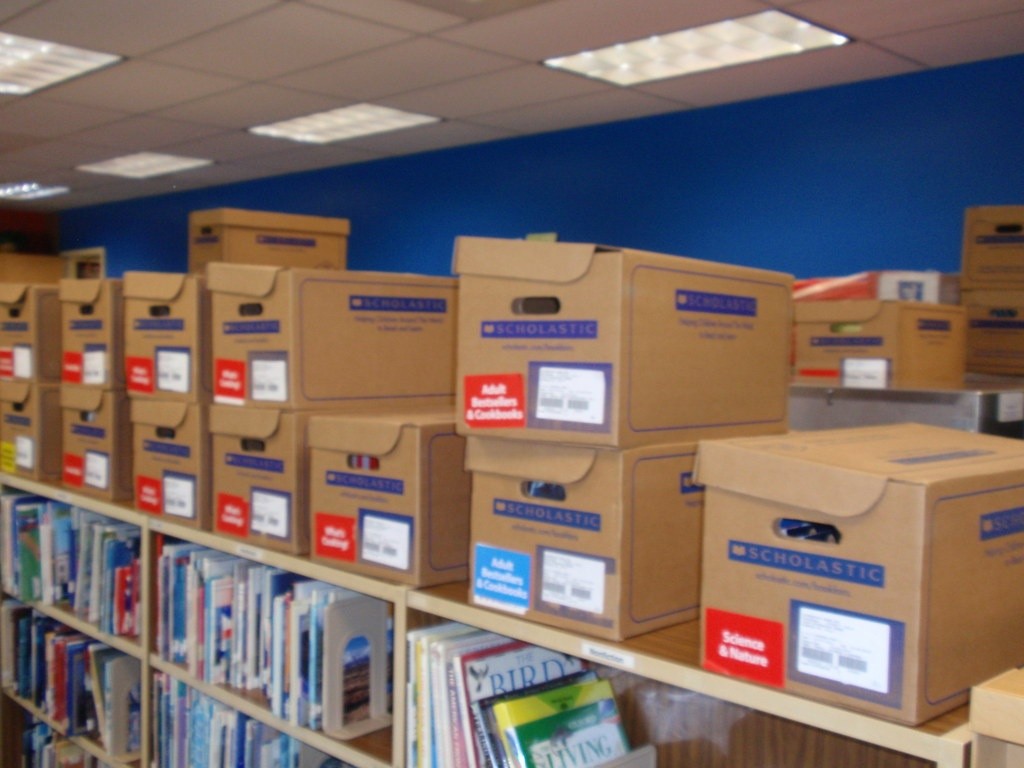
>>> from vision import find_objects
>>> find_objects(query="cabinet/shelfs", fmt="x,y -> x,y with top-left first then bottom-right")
0,468 -> 985,767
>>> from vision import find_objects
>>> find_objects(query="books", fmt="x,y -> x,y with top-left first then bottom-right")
0,485 -> 396,768
403,622 -> 632,768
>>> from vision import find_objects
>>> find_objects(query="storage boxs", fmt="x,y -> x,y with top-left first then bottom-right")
207,406 -> 313,557
123,269 -> 212,401
0,251 -> 70,285
305,408 -> 472,589
0,381 -> 61,482
56,278 -> 124,389
128,399 -> 215,530
790,204 -> 1024,392
462,437 -> 781,643
57,387 -> 132,503
691,421 -> 1023,727
206,261 -> 460,416
186,206 -> 348,277
451,235 -> 794,451
0,283 -> 62,381
